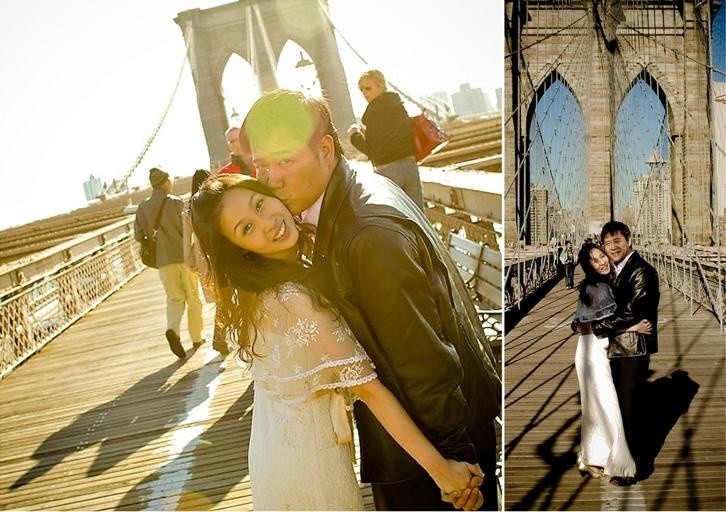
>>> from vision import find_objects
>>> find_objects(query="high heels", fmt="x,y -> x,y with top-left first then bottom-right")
578,462 -> 606,479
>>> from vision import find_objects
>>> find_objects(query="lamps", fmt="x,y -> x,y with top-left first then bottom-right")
295,50 -> 316,91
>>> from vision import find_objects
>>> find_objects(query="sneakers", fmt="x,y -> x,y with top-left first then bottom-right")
165,329 -> 230,358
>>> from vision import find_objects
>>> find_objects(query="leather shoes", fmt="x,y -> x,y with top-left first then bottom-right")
610,476 -> 648,486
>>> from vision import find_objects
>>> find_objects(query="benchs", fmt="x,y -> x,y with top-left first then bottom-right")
446,232 -> 501,364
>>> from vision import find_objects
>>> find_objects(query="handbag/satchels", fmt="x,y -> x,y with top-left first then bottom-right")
410,112 -> 451,166
139,231 -> 158,269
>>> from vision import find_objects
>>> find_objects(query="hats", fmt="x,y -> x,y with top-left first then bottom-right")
150,168 -> 170,188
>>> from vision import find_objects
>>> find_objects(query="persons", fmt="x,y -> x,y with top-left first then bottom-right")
216,125 -> 258,179
569,243 -> 655,488
133,165 -> 208,361
559,241 -> 577,290
345,69 -> 425,214
235,87 -> 501,510
568,219 -> 662,475
187,173 -> 490,510
181,168 -> 251,358
